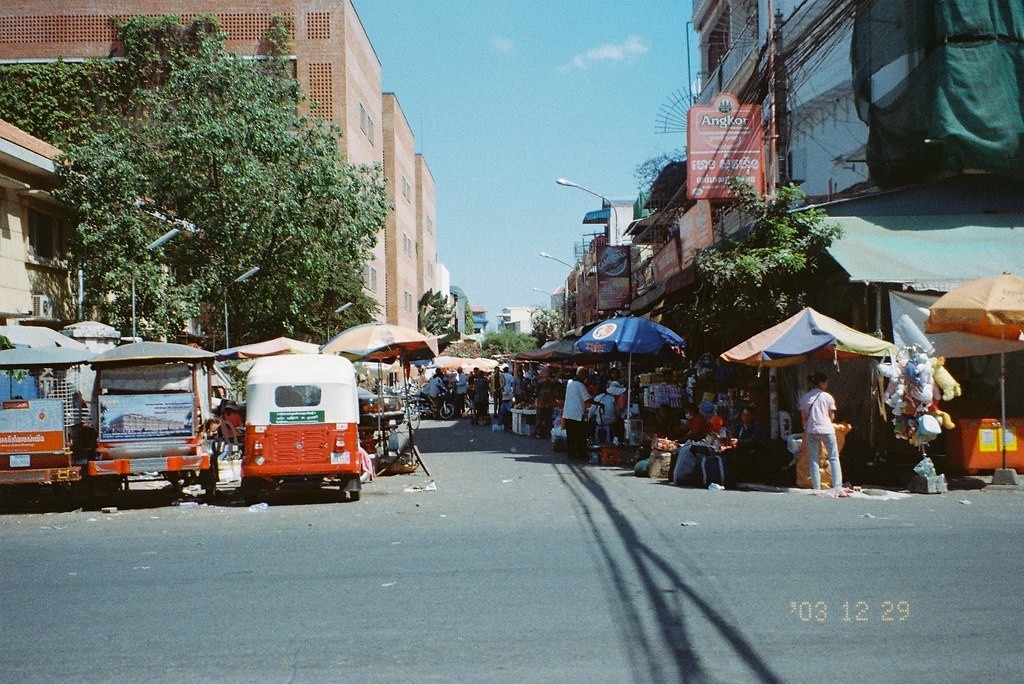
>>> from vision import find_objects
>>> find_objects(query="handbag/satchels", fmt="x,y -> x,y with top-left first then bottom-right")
696,455 -> 735,489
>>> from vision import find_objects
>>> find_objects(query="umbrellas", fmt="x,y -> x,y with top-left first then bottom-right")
430,356 -> 499,371
720,305 -> 899,391
516,314 -> 686,445
319,323 -> 438,454
924,271 -> 1024,470
216,337 -> 324,360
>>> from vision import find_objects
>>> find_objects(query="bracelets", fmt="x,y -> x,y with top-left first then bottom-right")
90,447 -> 94,451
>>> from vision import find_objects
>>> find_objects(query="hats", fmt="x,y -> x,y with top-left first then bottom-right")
606,381 -> 626,395
225,400 -> 239,411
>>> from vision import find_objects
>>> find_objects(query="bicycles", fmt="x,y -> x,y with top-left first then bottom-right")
388,382 -> 456,430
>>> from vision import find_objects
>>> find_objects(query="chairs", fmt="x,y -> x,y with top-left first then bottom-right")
217,421 -> 244,460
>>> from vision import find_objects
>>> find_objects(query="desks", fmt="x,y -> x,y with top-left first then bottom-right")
511,408 -> 536,435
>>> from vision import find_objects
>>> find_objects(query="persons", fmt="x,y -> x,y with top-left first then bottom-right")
220,404 -> 244,454
417,364 -> 761,485
72,416 -> 98,462
798,372 -> 850,497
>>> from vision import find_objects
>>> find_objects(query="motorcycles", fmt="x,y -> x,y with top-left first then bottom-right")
240,353 -> 361,502
85,342 -> 220,497
0,345 -> 91,503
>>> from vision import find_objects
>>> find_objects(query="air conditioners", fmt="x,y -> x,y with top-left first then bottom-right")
33,295 -> 53,317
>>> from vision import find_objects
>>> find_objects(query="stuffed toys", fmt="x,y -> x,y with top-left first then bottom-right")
878,343 -> 962,446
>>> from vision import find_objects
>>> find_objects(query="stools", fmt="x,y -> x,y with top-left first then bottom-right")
697,453 -> 725,487
595,426 -> 610,444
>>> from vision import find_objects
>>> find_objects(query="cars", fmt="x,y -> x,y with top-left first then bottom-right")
356,386 -> 405,436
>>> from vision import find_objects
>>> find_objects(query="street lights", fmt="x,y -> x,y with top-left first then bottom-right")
540,253 -> 580,327
224,266 -> 259,348
130,229 -> 180,342
555,178 -> 620,247
533,287 -> 561,319
326,300 -> 353,348
